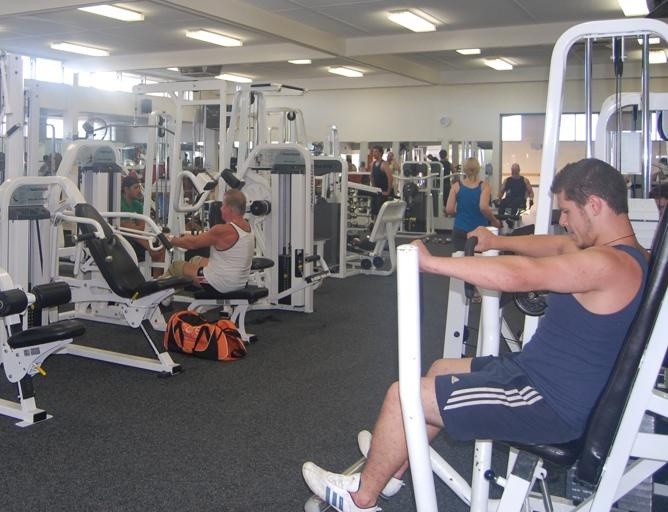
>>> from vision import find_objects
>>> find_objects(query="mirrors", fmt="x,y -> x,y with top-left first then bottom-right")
314,141 -> 495,219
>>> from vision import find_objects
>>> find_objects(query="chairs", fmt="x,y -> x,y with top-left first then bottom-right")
501,204 -> 668,485
75,204 -> 193,299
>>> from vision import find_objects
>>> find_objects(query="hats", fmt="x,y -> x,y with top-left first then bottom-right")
122,175 -> 140,186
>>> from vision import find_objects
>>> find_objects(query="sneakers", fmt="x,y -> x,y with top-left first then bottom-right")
302,461 -> 379,512
357,429 -> 404,502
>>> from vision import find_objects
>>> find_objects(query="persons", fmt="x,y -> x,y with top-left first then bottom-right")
648,187 -> 668,214
346,144 -> 535,253
38,151 -> 255,298
650,158 -> 668,187
302,158 -> 650,512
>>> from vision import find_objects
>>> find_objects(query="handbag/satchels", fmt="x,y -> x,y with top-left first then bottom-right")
163,310 -> 246,363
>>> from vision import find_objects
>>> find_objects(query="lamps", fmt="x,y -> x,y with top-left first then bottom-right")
618,0 -> 664,45
648,49 -> 667,64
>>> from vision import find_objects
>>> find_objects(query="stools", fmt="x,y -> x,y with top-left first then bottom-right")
7,320 -> 86,350
251,257 -> 275,272
194,286 -> 269,344
